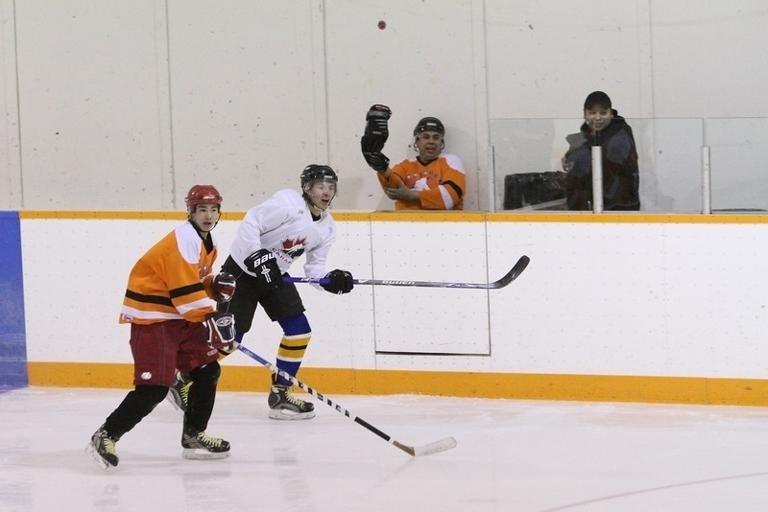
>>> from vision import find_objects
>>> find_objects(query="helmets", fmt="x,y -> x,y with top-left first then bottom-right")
184,184 -> 223,233
413,116 -> 446,137
299,163 -> 339,213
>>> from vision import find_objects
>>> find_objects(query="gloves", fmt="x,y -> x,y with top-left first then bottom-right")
243,248 -> 282,298
321,268 -> 355,296
363,103 -> 392,144
359,137 -> 389,173
211,271 -> 237,305
188,317 -> 238,366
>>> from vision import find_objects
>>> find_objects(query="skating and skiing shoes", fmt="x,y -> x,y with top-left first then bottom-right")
178,429 -> 233,461
265,384 -> 316,424
164,366 -> 193,419
82,422 -> 124,473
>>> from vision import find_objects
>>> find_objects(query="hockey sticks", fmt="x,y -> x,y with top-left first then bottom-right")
233,340 -> 457,457
282,254 -> 530,290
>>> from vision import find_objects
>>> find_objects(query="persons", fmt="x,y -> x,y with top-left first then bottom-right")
561,91 -> 641,211
86,183 -> 232,469
360,101 -> 466,211
164,163 -> 354,423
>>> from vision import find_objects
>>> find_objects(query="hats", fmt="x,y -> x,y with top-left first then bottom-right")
584,90 -> 612,110
